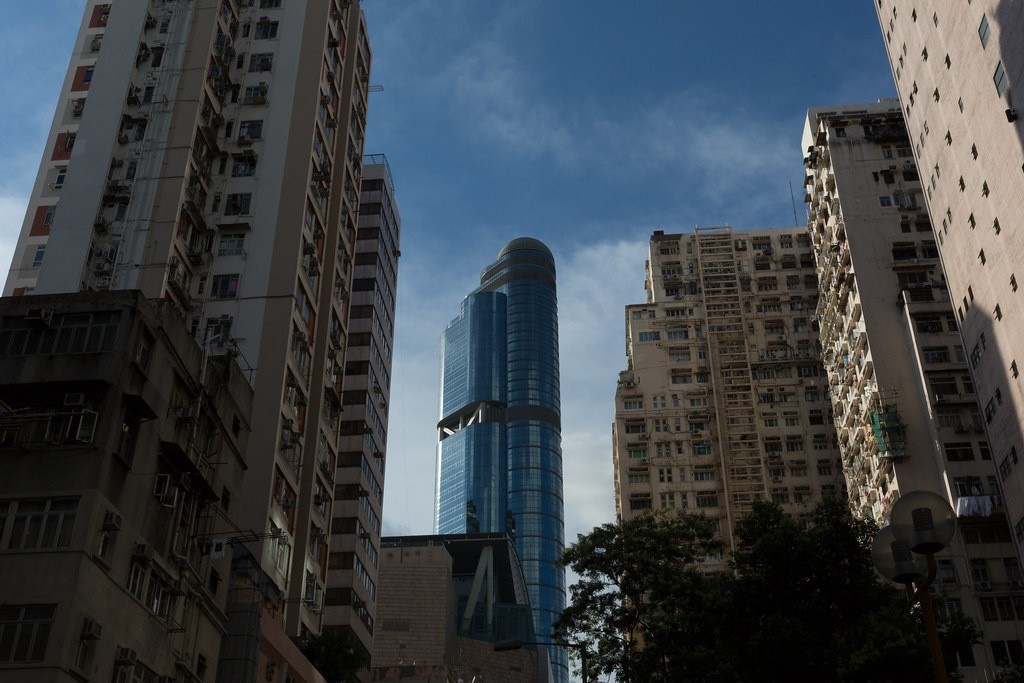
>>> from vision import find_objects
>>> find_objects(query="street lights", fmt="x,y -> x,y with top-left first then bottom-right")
495,640 -> 587,683
871,489 -> 958,683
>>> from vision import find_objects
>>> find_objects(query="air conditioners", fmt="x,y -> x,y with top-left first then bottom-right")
102,4 -> 111,14
772,476 -> 783,482
91,39 -> 101,51
333,11 -> 342,20
769,451 -> 779,457
361,73 -> 368,83
779,386 -> 785,392
148,17 -> 157,28
777,335 -> 787,342
135,543 -> 154,562
103,512 -> 123,531
63,392 -> 88,406
119,648 -> 137,665
313,494 -> 323,506
330,38 -> 339,49
328,72 -> 335,82
297,332 -> 306,341
754,252 -> 762,257
625,382 -> 634,389
94,215 -> 105,231
282,429 -> 295,450
93,262 -> 109,272
327,118 -> 338,128
220,314 -> 231,323
639,434 -> 649,439
693,429 -> 701,435
767,387 -> 775,393
101,15 -> 108,22
320,94 -> 330,106
305,243 -> 315,254
24,307 -> 50,328
82,622 -> 103,640
238,135 -> 251,144
640,457 -> 649,465
121,133 -> 128,144
260,16 -> 270,23
